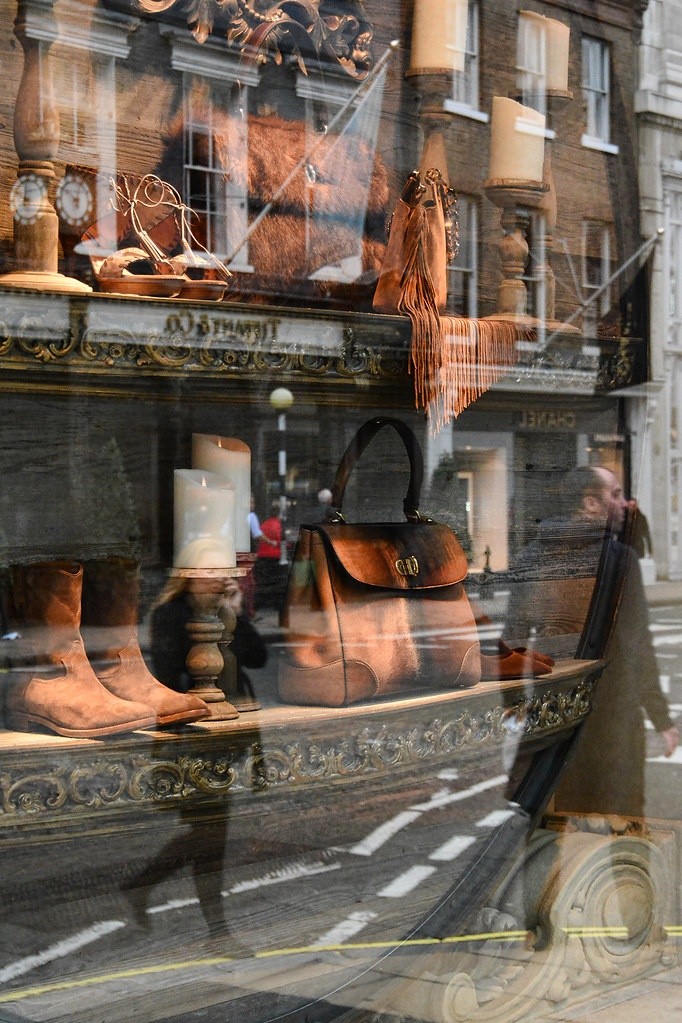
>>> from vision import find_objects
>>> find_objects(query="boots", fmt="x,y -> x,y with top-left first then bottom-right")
0,557 -> 155,739
80,558 -> 210,729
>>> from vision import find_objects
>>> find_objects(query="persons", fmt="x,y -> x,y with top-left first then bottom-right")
304,489 -> 346,524
252,505 -> 291,628
119,576 -> 253,959
226,494 -> 277,550
498,467 -> 680,952
616,498 -> 654,561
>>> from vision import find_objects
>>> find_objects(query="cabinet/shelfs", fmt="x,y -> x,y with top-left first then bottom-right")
1,0 -> 657,1022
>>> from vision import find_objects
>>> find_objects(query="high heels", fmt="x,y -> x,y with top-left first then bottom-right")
53,163 -> 185,298
117,170 -> 232,303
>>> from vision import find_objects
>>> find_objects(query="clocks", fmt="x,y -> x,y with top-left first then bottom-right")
55,175 -> 93,226
9,175 -> 47,226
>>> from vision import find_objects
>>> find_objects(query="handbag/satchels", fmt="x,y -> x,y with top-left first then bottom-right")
275,414 -> 482,706
637,557 -> 657,586
371,166 -> 545,420
206,18 -> 392,313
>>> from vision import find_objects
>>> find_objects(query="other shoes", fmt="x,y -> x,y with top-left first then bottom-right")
209,935 -> 256,961
478,638 -> 554,681
527,922 -> 567,950
628,924 -> 667,945
119,881 -> 153,932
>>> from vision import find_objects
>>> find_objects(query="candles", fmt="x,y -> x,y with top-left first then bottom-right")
172,468 -> 237,569
190,432 -> 251,555
515,10 -> 569,92
409,0 -> 468,71
490,96 -> 545,182
